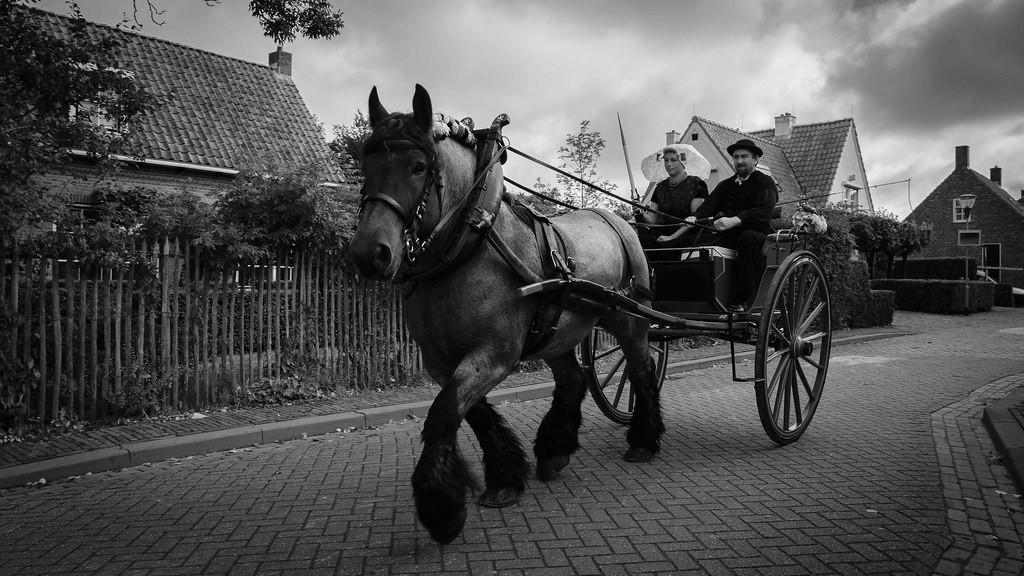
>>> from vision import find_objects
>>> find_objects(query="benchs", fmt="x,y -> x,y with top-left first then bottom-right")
677,206 -> 782,258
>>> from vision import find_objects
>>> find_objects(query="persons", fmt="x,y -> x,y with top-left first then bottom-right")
680,139 -> 779,312
631,144 -> 709,244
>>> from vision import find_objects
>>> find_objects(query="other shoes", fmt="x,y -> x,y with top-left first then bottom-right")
727,300 -> 750,311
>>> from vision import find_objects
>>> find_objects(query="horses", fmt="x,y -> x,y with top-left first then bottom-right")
343,84 -> 669,546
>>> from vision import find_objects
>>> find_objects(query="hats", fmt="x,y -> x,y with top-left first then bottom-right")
727,139 -> 763,157
641,144 -> 711,183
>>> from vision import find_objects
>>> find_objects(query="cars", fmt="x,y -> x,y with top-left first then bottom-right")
976,270 -> 1024,307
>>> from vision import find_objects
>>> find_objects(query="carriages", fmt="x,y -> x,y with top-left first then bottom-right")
351,84 -> 834,547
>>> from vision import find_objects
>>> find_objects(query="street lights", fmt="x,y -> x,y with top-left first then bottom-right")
959,190 -> 978,316
